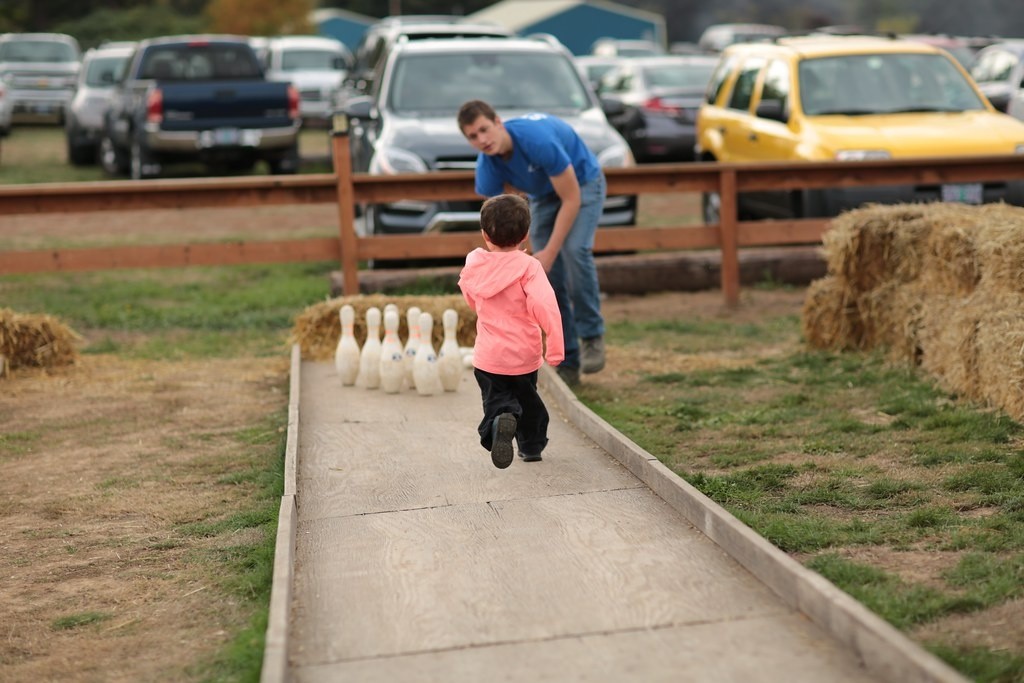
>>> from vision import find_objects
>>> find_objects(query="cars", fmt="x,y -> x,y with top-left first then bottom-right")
0,32 -> 82,127
248,36 -> 357,129
330,33 -> 635,270
331,14 -> 514,107
66,41 -> 140,168
575,22 -> 1024,204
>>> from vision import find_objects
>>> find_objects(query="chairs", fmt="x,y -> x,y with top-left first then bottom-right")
876,65 -> 919,108
803,69 -> 822,112
213,57 -> 232,76
152,60 -> 174,78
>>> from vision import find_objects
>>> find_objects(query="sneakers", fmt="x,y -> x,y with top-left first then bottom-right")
518,449 -> 543,463
490,413 -> 517,468
554,364 -> 580,386
579,336 -> 606,375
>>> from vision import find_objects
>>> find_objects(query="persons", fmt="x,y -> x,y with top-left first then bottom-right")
456,194 -> 564,469
458,100 -> 606,388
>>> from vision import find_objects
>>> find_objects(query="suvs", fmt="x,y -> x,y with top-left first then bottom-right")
694,31 -> 1024,227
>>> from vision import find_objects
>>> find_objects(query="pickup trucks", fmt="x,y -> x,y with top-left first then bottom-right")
101,35 -> 302,180
